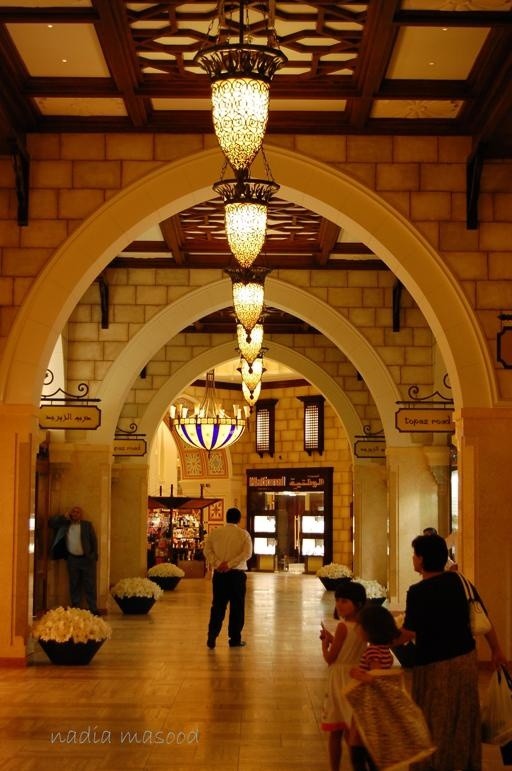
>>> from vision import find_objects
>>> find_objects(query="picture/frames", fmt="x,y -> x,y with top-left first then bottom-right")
207,498 -> 225,521
207,523 -> 224,535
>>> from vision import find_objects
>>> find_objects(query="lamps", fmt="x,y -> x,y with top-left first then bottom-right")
171,369 -> 247,459
295,394 -> 326,455
194,0 -> 289,409
254,399 -> 279,458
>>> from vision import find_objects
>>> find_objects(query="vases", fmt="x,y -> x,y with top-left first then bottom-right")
151,576 -> 180,592
365,598 -> 385,609
39,636 -> 106,667
114,596 -> 154,616
319,576 -> 350,592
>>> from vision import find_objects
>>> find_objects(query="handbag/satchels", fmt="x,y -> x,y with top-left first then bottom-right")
481,666 -> 512,747
468,593 -> 492,637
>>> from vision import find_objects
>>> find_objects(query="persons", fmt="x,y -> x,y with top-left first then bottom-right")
46,505 -> 101,617
423,527 -> 454,571
390,534 -> 512,771
202,507 -> 253,649
318,582 -> 368,771
351,605 -> 401,771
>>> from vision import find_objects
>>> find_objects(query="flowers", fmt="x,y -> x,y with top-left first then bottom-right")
316,562 -> 355,579
33,603 -> 111,644
148,561 -> 186,579
110,576 -> 164,600
350,576 -> 387,599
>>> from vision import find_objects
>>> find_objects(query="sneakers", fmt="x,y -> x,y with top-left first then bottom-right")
228,639 -> 245,646
